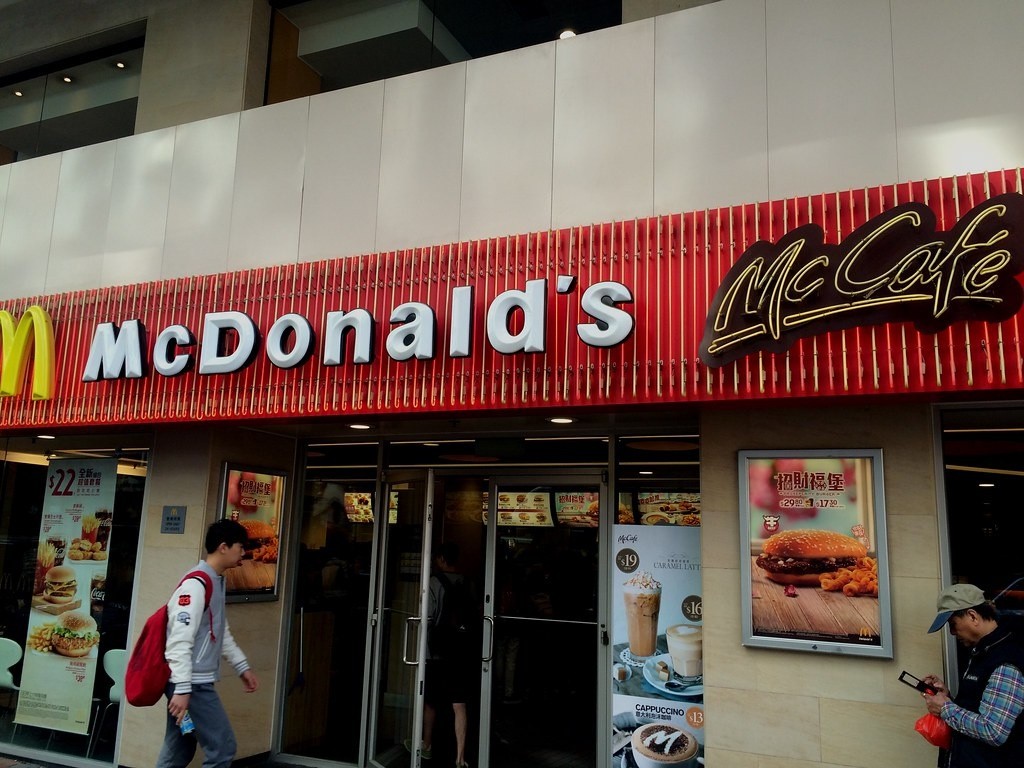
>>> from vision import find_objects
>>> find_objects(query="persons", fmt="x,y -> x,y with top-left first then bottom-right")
919,583 -> 1024,768
402,541 -> 524,767
162,518 -> 259,768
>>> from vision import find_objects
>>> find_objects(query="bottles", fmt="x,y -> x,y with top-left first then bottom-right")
168,688 -> 196,737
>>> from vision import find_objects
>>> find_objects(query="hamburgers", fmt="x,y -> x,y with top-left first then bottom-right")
53,611 -> 99,657
239,519 -> 275,558
43,566 -> 77,603
499,494 -> 547,522
756,530 -> 868,585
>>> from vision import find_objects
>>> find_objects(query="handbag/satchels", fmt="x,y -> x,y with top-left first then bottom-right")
915,689 -> 950,749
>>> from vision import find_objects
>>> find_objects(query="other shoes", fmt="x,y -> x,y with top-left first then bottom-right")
405,739 -> 432,760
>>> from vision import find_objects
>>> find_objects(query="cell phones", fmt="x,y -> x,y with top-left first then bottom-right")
897,670 -> 938,695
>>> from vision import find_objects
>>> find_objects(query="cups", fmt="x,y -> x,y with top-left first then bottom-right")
630,723 -> 704,768
623,587 -> 661,656
46,535 -> 67,566
94,512 -> 112,551
665,625 -> 702,676
90,577 -> 107,633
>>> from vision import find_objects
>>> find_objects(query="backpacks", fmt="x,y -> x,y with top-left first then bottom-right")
434,573 -> 486,646
125,570 -> 213,707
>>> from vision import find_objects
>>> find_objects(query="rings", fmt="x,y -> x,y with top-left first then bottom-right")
923,692 -> 926,697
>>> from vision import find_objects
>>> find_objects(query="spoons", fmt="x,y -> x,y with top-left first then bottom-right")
665,680 -> 702,691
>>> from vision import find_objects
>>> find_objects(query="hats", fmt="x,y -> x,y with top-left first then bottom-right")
927,584 -> 986,634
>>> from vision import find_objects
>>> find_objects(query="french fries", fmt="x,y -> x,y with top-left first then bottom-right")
82,515 -> 101,533
27,622 -> 55,653
38,542 -> 58,568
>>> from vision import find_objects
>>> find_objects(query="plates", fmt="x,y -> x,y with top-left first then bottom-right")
643,653 -> 703,695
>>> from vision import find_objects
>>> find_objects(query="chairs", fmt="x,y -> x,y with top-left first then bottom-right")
0,637 -> 127,758
